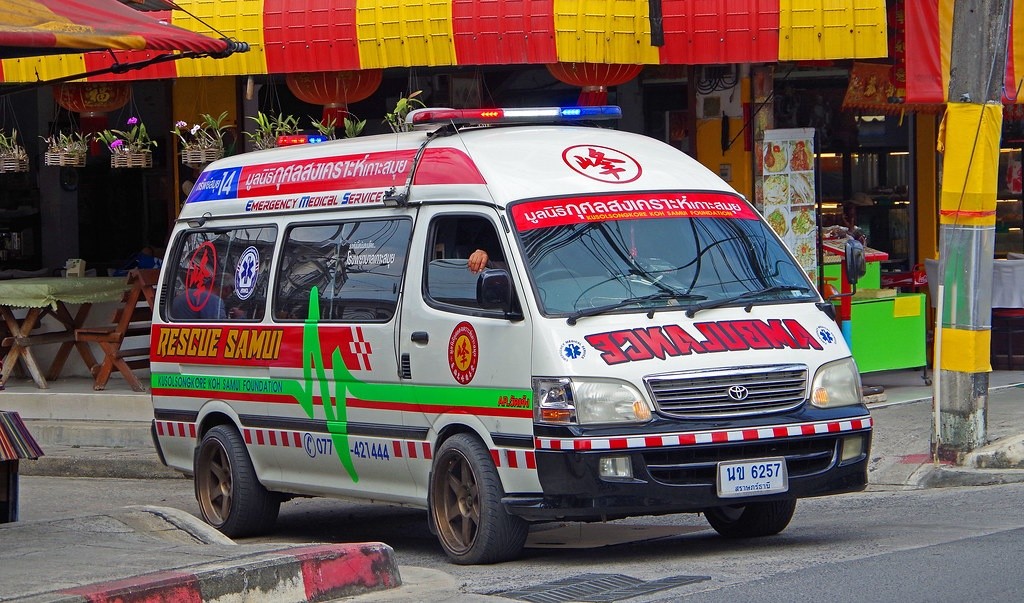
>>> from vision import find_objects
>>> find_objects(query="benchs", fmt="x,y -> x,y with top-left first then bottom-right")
74,267 -> 162,392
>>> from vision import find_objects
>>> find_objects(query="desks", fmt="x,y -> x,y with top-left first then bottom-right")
0,276 -> 129,389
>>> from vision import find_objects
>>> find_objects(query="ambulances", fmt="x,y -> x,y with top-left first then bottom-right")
150,106 -> 874,565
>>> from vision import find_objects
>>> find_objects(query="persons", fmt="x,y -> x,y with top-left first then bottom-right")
170,272 -> 245,319
467,249 -> 507,274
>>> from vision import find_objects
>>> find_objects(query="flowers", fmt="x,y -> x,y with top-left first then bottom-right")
169,110 -> 237,150
93,116 -> 158,154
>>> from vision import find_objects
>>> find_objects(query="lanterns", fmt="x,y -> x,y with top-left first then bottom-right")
545,61 -> 644,106
53,81 -> 135,158
285,68 -> 383,130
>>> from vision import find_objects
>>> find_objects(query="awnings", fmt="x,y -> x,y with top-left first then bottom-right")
0,0 -> 1024,106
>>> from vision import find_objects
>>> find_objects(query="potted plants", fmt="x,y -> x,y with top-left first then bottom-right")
241,110 -> 303,152
0,128 -> 30,174
39,133 -> 92,168
